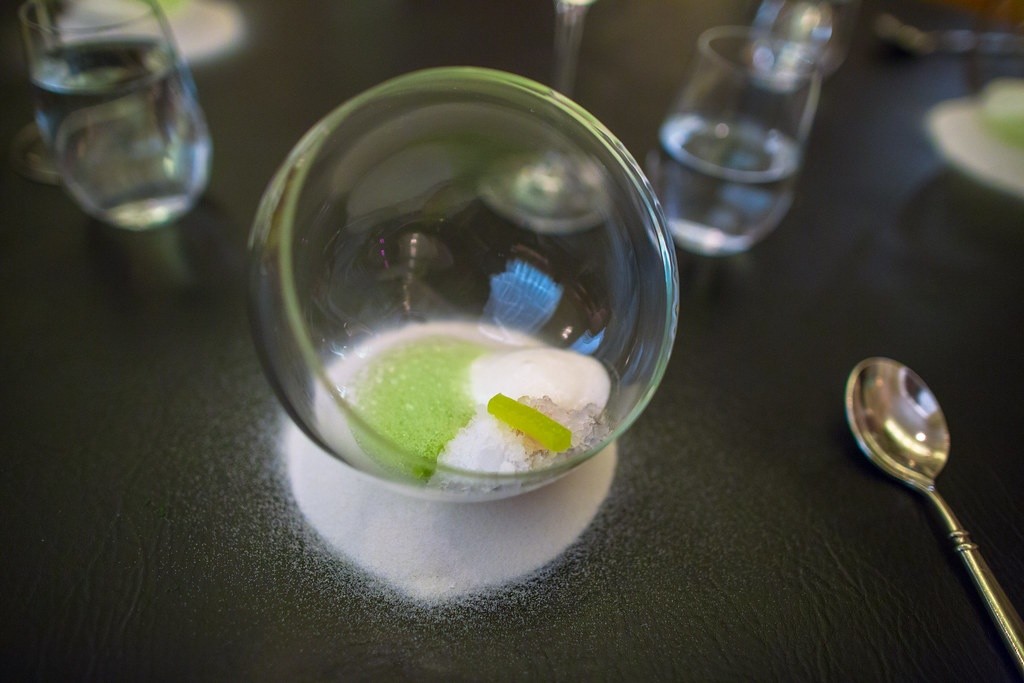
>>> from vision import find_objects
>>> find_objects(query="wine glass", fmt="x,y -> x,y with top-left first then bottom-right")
469,0 -> 611,233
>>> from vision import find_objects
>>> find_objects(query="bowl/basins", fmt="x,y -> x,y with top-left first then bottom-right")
244,66 -> 681,504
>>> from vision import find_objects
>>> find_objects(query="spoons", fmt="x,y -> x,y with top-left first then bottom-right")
845,357 -> 1024,676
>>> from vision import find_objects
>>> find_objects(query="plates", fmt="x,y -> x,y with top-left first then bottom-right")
924,101 -> 1024,195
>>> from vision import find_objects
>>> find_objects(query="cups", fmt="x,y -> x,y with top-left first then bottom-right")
19,1 -> 212,230
644,27 -> 821,257
